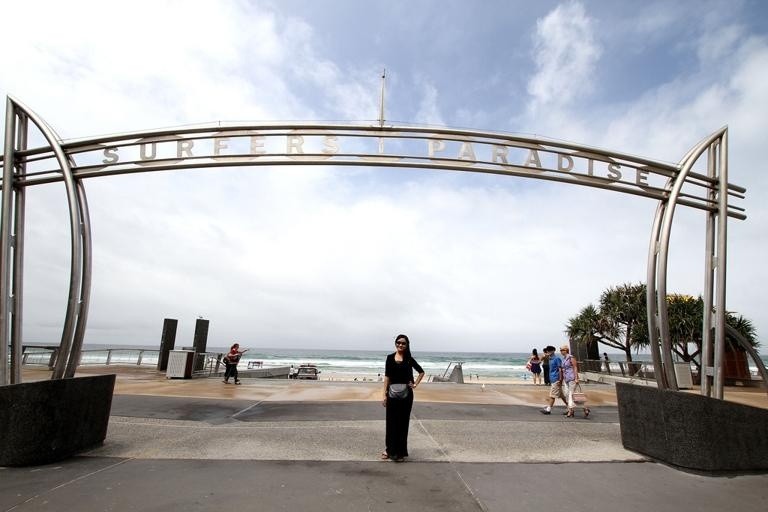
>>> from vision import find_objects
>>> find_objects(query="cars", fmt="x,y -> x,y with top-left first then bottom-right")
295,367 -> 320,379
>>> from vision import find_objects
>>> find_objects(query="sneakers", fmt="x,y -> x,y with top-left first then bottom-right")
539,408 -> 549,415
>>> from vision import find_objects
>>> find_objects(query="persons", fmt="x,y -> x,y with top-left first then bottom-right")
601,351 -> 611,374
379,334 -> 425,463
289,365 -> 295,379
529,348 -> 542,386
560,344 -> 592,418
224,346 -> 241,382
221,343 -> 243,385
539,346 -> 569,415
540,347 -> 552,386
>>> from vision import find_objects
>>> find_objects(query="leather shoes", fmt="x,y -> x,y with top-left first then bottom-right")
563,410 -> 567,415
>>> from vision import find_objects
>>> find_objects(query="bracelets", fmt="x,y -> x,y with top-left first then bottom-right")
414,384 -> 417,387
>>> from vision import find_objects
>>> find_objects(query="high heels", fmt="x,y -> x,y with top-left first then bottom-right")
565,410 -> 574,417
583,407 -> 590,417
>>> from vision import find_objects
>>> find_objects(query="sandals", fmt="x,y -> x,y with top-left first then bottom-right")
381,451 -> 387,459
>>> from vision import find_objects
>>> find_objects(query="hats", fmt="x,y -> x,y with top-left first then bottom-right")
545,346 -> 555,351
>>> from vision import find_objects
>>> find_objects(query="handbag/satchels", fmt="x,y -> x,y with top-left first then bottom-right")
572,383 -> 586,403
230,356 -> 238,362
387,383 -> 410,399
526,362 -> 531,370
